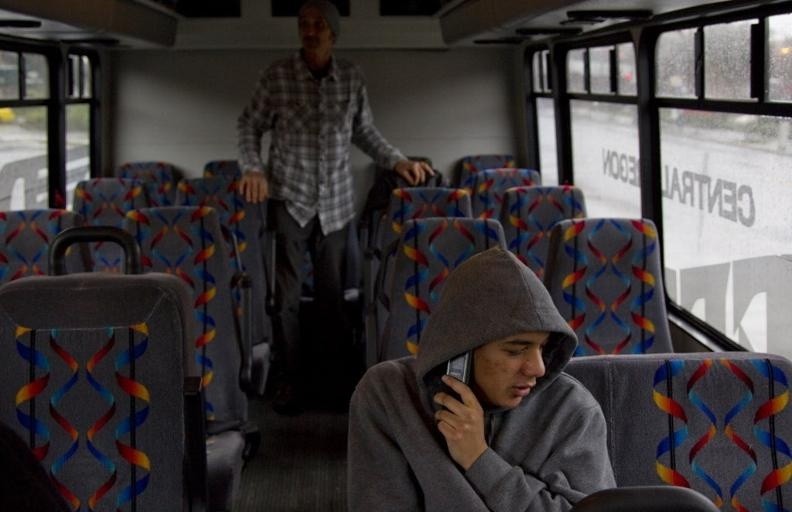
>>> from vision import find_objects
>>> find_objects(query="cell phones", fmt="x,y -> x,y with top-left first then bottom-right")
442,351 -> 472,413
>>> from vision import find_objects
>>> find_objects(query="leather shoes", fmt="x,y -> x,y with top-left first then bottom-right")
271,377 -> 299,415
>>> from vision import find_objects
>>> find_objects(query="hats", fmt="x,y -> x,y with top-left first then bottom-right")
299,2 -> 340,44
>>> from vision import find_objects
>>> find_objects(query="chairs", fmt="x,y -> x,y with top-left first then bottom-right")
349,150 -> 790,511
0,160 -> 286,512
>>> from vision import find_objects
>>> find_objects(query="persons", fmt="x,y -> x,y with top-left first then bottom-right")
345,247 -> 617,511
236,0 -> 437,412
